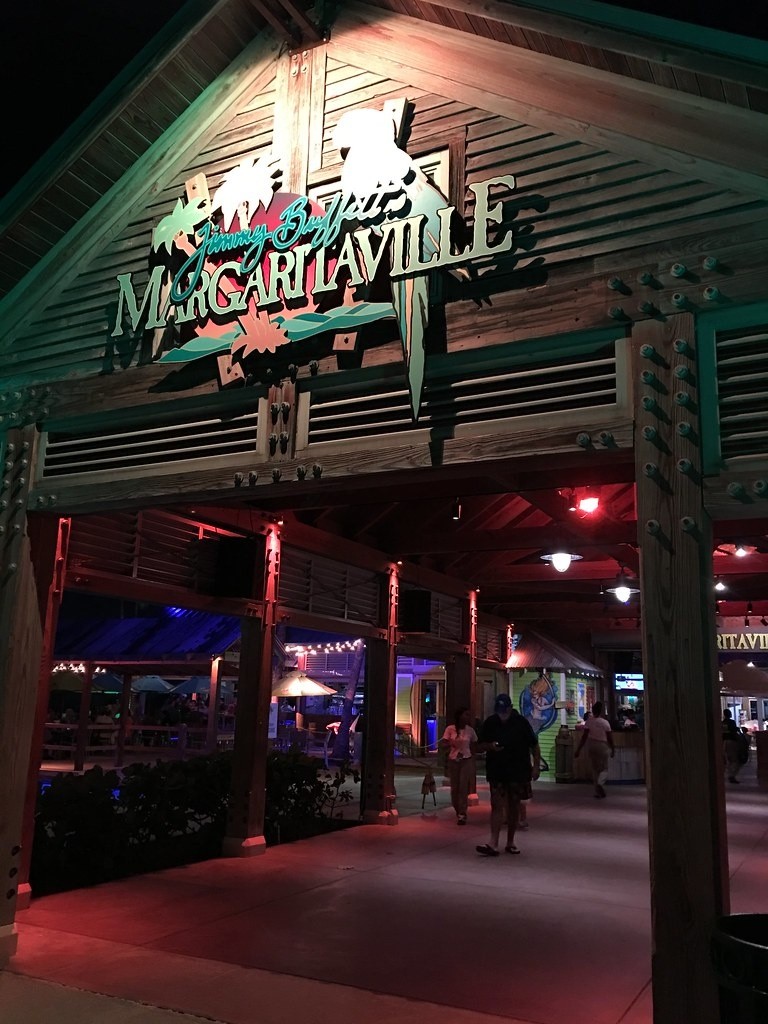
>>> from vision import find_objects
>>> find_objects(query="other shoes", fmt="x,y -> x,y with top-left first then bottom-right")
596,784 -> 606,798
593,793 -> 601,798
520,819 -> 529,828
457,813 -> 467,825
728,776 -> 740,784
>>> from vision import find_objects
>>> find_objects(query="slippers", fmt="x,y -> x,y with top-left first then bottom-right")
506,846 -> 520,854
476,844 -> 500,856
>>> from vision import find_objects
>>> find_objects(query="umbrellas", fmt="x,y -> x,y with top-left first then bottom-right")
49,668 -> 338,729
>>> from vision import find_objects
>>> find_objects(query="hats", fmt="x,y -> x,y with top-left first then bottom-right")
494,693 -> 511,712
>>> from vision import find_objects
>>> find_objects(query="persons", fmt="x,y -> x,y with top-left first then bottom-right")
574,699 -> 752,798
442,707 -> 488,826
44,693 -> 236,745
478,693 -> 541,857
